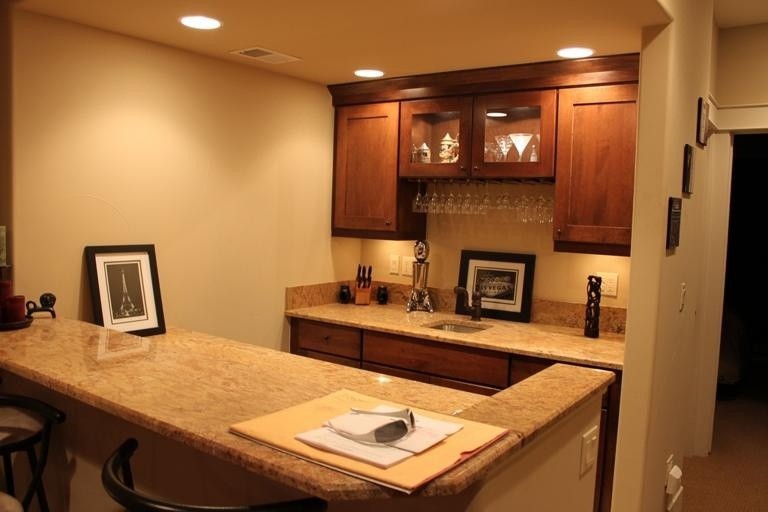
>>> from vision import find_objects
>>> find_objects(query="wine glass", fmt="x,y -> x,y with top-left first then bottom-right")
412,177 -> 553,225
488,130 -> 538,162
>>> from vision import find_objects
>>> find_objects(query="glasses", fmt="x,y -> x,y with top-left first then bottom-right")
326,407 -> 417,445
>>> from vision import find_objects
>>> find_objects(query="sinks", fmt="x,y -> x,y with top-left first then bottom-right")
420,318 -> 494,335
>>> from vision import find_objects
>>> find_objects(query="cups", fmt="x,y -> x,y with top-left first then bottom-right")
5,296 -> 26,323
0,279 -> 14,322
340,286 -> 351,302
378,286 -> 388,304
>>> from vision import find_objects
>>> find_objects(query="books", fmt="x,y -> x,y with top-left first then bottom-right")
230,386 -> 509,497
295,404 -> 465,470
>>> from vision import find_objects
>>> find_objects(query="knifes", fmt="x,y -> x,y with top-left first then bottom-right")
356,264 -> 373,288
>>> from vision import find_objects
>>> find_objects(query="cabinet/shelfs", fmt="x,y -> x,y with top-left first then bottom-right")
549,53 -> 641,253
395,59 -> 556,187
322,75 -> 429,243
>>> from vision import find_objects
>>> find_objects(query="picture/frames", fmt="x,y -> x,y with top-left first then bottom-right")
681,141 -> 696,196
80,243 -> 169,340
664,196 -> 682,252
453,247 -> 537,324
694,94 -> 713,149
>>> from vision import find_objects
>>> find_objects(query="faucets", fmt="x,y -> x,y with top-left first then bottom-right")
453,286 -> 483,322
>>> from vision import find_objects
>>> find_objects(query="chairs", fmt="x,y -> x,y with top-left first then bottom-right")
94,433 -> 328,512
0,389 -> 66,512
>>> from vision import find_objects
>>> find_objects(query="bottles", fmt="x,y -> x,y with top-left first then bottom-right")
410,141 -> 430,163
440,132 -> 459,164
